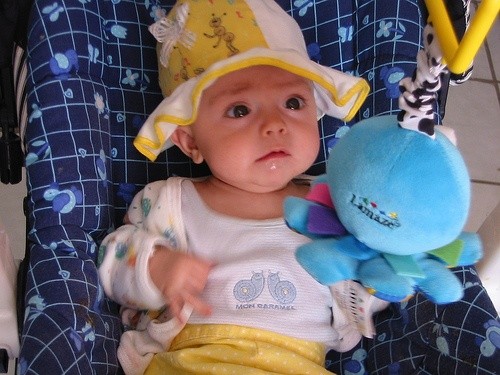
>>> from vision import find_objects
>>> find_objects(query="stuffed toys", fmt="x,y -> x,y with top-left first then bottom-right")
282,112 -> 485,306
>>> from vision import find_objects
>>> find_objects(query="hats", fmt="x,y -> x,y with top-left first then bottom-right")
133,0 -> 370,161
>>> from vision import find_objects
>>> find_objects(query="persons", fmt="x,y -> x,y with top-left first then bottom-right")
94,0 -> 385,375
